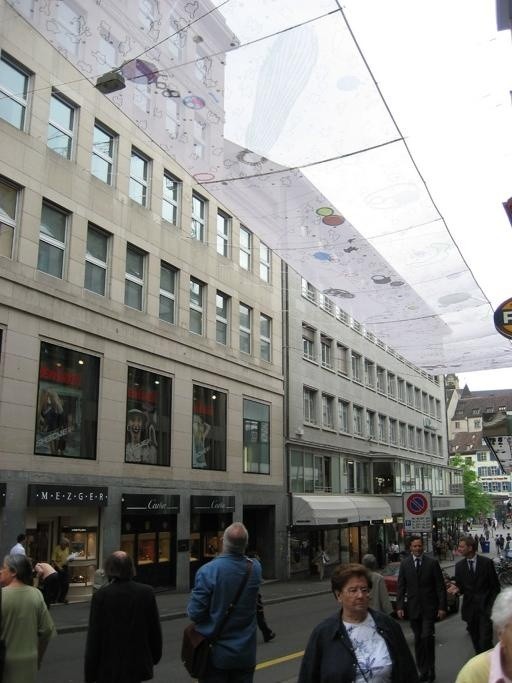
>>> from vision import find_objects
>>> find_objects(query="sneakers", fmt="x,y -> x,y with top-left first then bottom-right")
57,598 -> 68,603
419,673 -> 436,682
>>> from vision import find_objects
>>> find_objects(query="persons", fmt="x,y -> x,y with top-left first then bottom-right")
36,386 -> 75,456
83,548 -> 163,682
0,532 -> 71,683
248,549 -> 276,642
126,402 -> 158,465
361,553 -> 392,617
446,536 -> 500,655
296,561 -> 420,683
395,535 -> 447,682
193,415 -> 212,467
186,523 -> 261,683
376,538 -> 400,570
307,543 -> 329,581
454,587 -> 511,682
432,512 -> 512,563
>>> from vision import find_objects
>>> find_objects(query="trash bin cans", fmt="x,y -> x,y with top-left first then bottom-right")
482,542 -> 489,553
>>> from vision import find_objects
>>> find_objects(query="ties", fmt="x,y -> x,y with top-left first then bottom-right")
468,560 -> 475,577
414,557 -> 421,572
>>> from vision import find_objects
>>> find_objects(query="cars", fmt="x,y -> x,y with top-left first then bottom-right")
377,558 -> 461,625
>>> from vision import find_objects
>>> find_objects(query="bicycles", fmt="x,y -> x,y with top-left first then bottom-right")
493,548 -> 512,588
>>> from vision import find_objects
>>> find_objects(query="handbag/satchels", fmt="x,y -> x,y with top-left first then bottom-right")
181,624 -> 211,679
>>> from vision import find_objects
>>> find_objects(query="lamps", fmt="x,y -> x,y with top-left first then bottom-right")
93,67 -> 127,94
